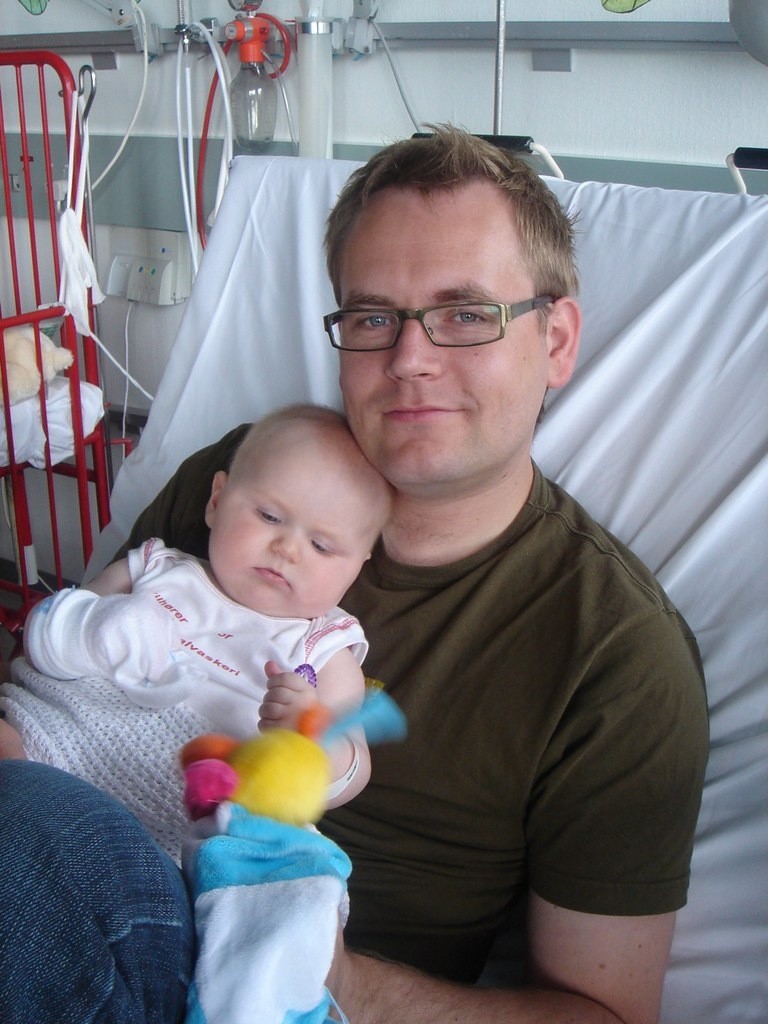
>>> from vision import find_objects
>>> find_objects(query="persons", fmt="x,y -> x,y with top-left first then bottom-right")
0,405 -> 395,885
0,120 -> 711,1021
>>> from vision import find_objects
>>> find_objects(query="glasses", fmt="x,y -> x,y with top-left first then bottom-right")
324,294 -> 554,351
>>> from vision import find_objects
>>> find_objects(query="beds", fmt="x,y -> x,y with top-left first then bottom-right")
81,153 -> 768,1024
0,48 -> 134,665
